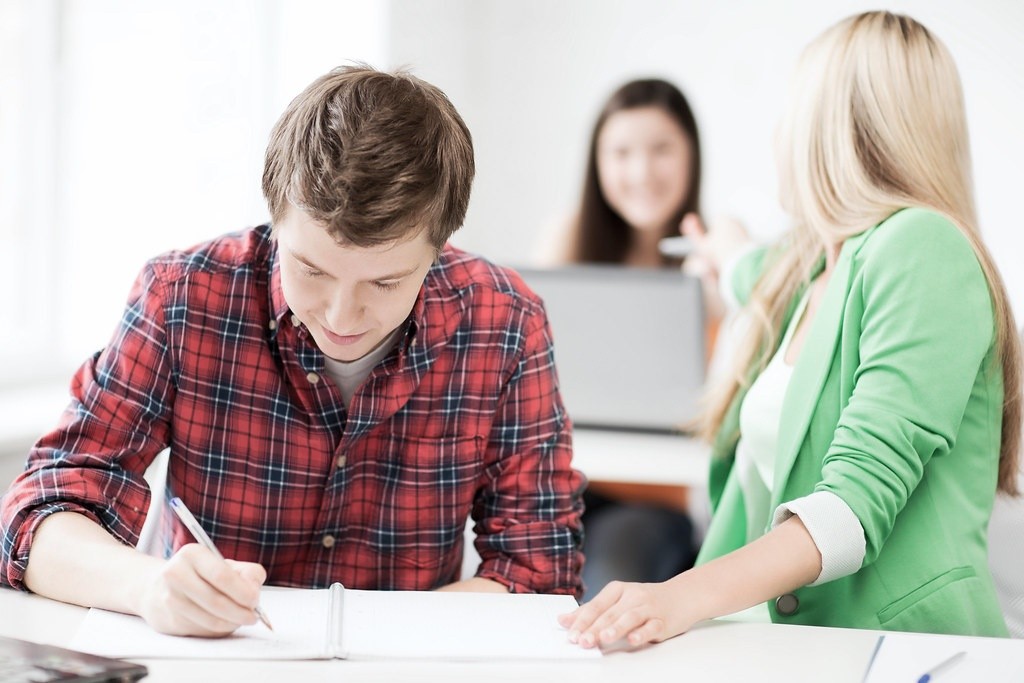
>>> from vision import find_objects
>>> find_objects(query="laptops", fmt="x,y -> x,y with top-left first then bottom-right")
510,268 -> 708,437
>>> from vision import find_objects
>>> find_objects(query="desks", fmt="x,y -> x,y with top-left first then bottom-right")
1,582 -> 1024,683
570,424 -> 704,506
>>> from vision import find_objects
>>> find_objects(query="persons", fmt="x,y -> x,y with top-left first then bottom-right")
0,60 -> 586,638
563,11 -> 1024,649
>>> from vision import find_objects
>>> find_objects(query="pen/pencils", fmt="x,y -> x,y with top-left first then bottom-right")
163,489 -> 273,630
657,236 -> 744,254
918,652 -> 968,683
